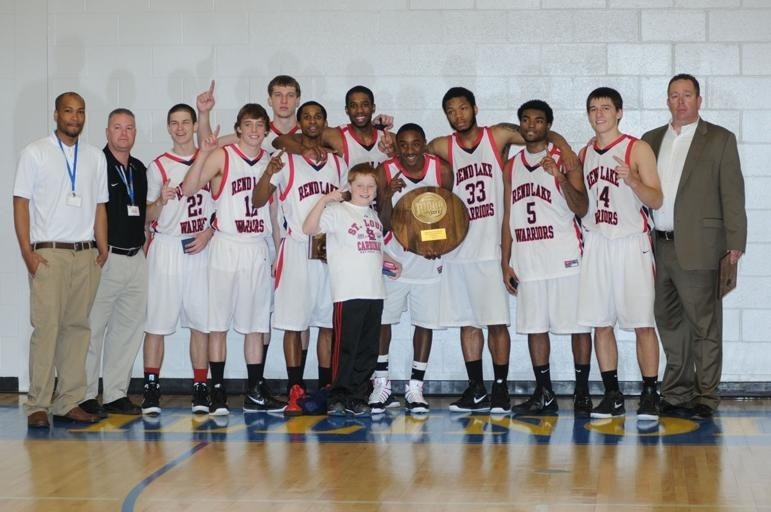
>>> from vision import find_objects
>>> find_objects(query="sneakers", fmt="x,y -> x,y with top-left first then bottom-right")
490,377 -> 512,414
635,387 -> 715,421
573,389 -> 593,419
140,381 -> 162,416
511,385 -> 559,416
284,383 -> 307,417
242,377 -> 289,413
327,376 -> 401,416
589,390 -> 627,420
191,381 -> 231,415
403,380 -> 430,413
448,387 -> 491,413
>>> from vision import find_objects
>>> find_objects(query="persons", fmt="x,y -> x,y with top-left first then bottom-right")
576,87 -> 664,421
641,74 -> 747,416
193,73 -> 310,399
270,85 -> 397,172
139,103 -> 210,416
300,161 -> 402,418
78,108 -> 148,418
250,100 -> 349,415
379,87 -> 580,415
181,103 -> 288,417
366,123 -> 454,415
499,99 -> 594,417
12,90 -> 110,428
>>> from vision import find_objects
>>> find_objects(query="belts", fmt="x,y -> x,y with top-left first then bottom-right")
655,229 -> 674,241
108,245 -> 141,257
30,241 -> 96,252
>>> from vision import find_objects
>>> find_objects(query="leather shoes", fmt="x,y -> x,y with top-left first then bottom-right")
52,406 -> 101,425
78,399 -> 109,419
28,409 -> 51,430
103,396 -> 142,416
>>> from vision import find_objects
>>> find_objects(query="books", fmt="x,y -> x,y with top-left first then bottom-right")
717,251 -> 737,301
